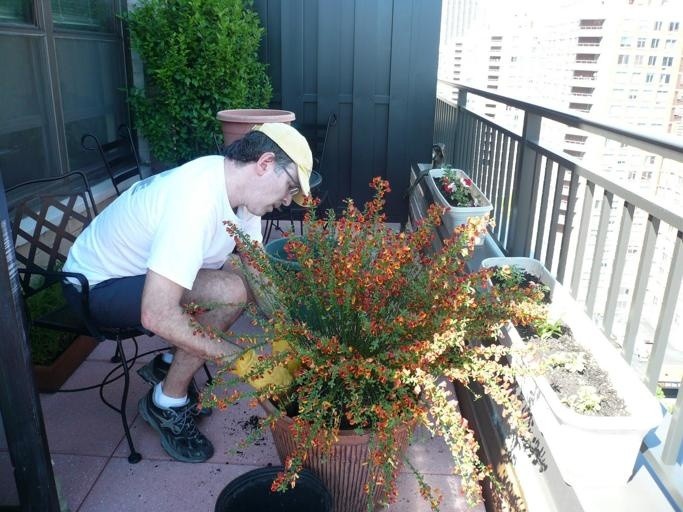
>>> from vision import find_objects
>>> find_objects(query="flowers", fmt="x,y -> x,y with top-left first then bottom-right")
442,173 -> 472,192
494,266 -> 546,302
189,175 -> 584,510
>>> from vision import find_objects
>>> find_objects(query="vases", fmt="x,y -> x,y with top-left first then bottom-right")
213,106 -> 298,159
263,230 -> 375,291
248,389 -> 423,507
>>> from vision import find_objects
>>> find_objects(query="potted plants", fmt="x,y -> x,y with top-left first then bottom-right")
22,266 -> 108,392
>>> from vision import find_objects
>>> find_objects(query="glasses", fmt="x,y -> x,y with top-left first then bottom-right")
275,154 -> 302,198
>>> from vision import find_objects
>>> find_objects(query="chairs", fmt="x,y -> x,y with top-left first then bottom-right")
2,171 -> 215,464
248,112 -> 339,247
77,124 -> 146,195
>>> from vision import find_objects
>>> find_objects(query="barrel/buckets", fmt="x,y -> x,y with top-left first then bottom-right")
263,236 -> 337,273
217,109 -> 297,147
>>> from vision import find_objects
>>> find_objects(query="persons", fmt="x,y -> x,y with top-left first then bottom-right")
60,123 -> 314,463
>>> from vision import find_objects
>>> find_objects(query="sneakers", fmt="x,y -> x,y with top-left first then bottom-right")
137,352 -> 213,416
137,386 -> 214,463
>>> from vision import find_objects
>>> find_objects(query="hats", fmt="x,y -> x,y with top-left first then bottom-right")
253,122 -> 318,208
